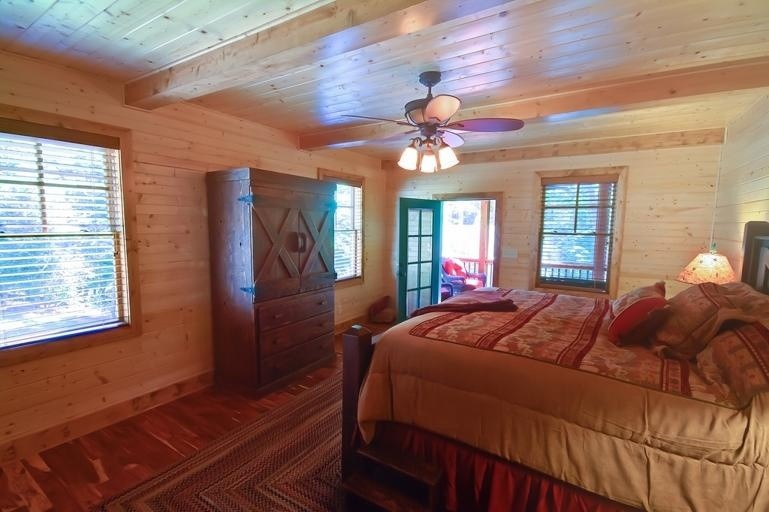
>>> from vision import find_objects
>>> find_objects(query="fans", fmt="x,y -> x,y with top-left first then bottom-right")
341,71 -> 524,148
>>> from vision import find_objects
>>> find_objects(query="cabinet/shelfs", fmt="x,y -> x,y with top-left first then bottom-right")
206,166 -> 336,401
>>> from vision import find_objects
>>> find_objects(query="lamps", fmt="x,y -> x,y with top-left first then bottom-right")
398,128 -> 460,172
675,242 -> 736,285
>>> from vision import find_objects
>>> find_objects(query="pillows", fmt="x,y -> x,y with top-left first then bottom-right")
649,283 -> 756,358
608,282 -> 671,347
697,283 -> 769,409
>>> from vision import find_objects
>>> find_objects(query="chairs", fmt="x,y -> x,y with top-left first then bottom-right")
441,257 -> 486,301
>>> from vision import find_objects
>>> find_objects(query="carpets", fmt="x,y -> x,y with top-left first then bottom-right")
98,369 -> 346,511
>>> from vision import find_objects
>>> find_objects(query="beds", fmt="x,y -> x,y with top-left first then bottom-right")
342,220 -> 769,512
